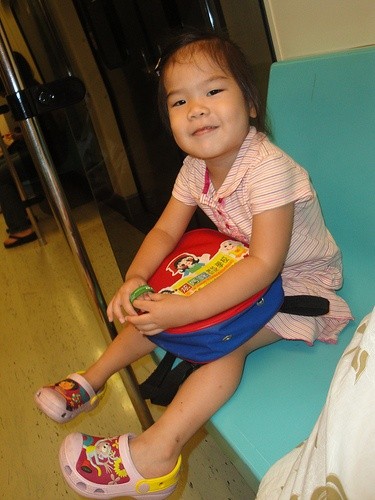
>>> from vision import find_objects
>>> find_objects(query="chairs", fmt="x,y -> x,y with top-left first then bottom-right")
99,194 -> 198,283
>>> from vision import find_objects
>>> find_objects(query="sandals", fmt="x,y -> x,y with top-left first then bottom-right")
34,371 -> 107,424
58,432 -> 182,499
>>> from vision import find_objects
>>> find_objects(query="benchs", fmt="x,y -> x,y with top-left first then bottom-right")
153,45 -> 375,498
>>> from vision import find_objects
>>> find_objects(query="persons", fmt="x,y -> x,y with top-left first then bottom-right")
251,306 -> 375,500
32,29 -> 356,500
